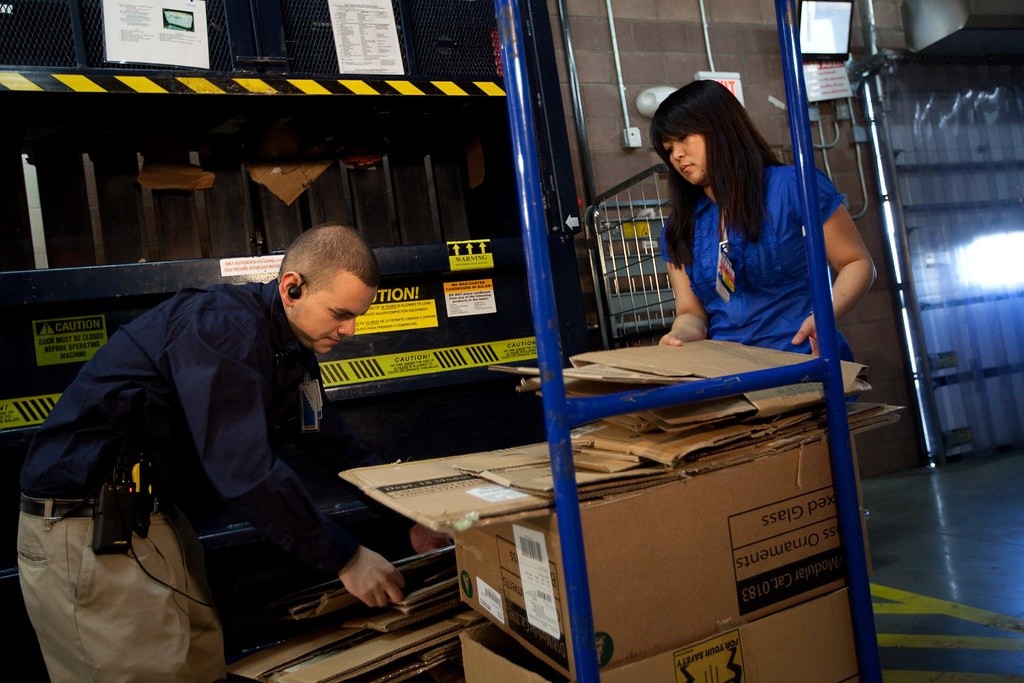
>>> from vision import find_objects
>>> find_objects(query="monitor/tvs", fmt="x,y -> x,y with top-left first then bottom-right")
797,0 -> 853,62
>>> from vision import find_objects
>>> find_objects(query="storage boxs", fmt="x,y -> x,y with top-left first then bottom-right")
456,430 -> 874,683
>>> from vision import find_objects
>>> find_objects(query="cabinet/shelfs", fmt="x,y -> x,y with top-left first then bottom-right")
0,0 -> 592,683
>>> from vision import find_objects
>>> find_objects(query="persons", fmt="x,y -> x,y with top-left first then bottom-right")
16,221 -> 456,683
648,79 -> 876,403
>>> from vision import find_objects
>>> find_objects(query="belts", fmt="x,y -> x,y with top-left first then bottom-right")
19,495 -> 93,517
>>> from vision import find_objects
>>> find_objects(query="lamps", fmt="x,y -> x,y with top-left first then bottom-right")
797,0 -> 854,63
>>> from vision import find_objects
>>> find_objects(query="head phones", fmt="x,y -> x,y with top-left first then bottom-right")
288,273 -> 305,299
